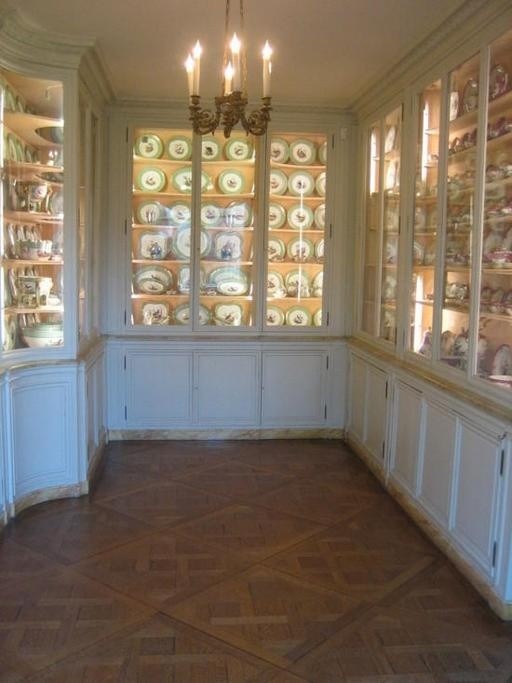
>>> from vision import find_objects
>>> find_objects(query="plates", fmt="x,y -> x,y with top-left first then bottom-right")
4,85 -> 75,351
130,126 -> 327,329
366,66 -> 512,383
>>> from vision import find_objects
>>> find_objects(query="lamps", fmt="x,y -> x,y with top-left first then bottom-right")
184,1 -> 273,139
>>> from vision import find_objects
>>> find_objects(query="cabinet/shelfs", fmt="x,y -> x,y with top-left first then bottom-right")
124,130 -> 334,429
347,27 -> 512,603
1,72 -> 100,359
